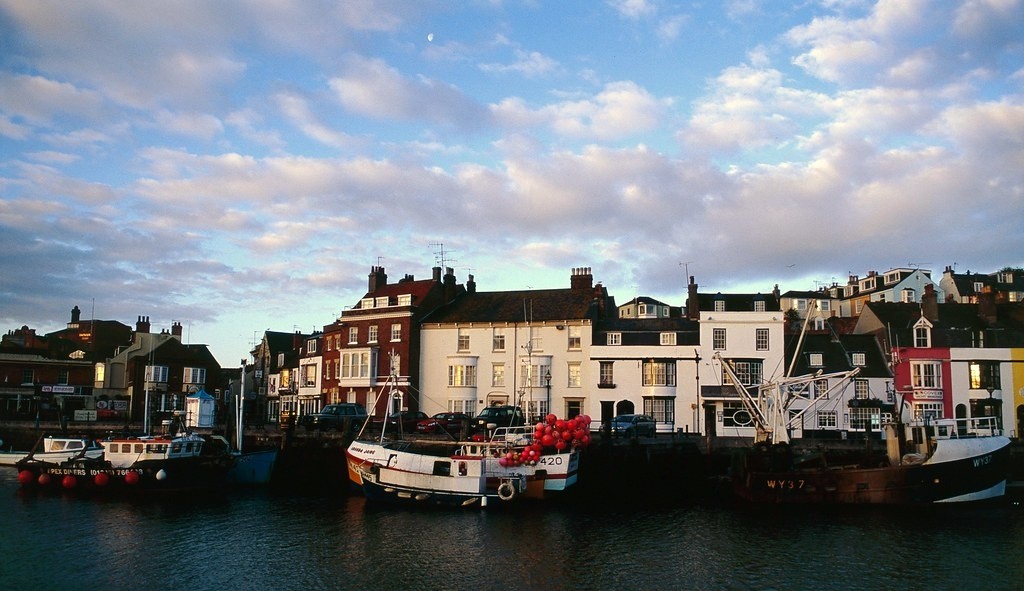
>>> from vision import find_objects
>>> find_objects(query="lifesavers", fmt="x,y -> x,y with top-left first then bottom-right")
497,483 -> 515,502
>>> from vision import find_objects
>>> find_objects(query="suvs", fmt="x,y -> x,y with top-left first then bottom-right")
301,402 -> 367,431
471,405 -> 526,436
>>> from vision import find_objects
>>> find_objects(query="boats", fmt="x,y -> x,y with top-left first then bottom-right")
0,358 -> 281,496
714,297 -> 1013,516
344,347 -> 581,509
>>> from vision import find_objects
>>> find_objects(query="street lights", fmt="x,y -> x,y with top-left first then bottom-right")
986,385 -> 995,416
544,369 -> 553,416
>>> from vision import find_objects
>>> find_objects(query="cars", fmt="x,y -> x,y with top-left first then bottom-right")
379,410 -> 428,434
416,412 -> 470,436
598,414 -> 656,438
